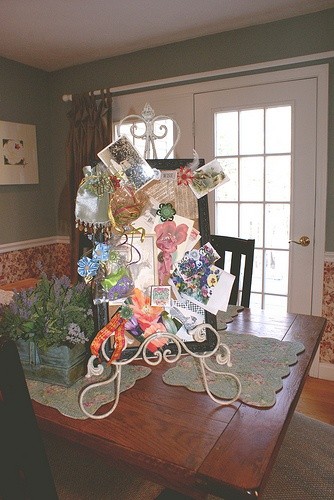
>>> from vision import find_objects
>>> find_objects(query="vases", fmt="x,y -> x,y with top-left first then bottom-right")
15,338 -> 88,388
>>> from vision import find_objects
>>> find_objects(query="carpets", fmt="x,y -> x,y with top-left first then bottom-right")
43,411 -> 334,500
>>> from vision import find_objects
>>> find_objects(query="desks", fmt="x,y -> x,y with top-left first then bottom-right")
1,279 -> 326,500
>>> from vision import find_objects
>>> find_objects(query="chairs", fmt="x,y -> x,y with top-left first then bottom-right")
0,340 -> 56,500
209,234 -> 255,308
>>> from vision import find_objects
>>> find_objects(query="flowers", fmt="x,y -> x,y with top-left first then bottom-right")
89,306 -> 133,363
156,232 -> 178,280
154,204 -> 178,222
77,244 -> 112,281
0,276 -> 94,347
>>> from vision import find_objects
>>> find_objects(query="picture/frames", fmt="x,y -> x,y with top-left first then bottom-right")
89,159 -> 218,361
